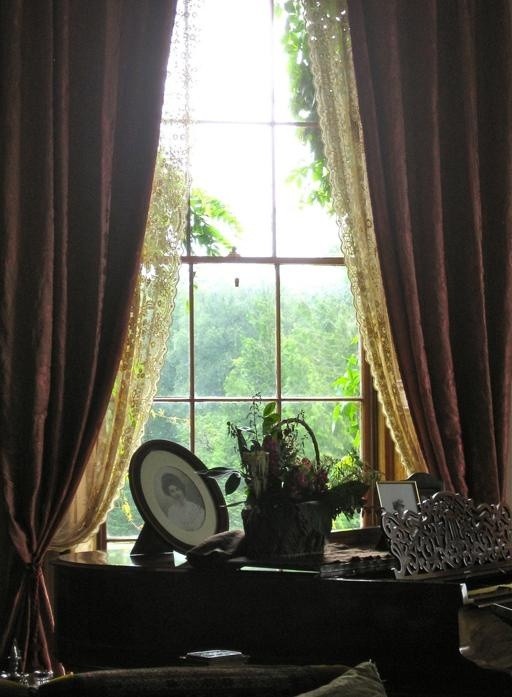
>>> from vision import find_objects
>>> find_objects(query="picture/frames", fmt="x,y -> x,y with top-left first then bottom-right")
374,480 -> 422,552
127,439 -> 230,557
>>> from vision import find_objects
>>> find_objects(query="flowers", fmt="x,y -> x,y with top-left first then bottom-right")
193,388 -> 386,522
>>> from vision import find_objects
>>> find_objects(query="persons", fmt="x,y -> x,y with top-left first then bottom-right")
390,500 -> 409,516
160,474 -> 204,528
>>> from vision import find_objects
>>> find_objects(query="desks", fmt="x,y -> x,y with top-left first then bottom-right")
51,546 -> 511,672
